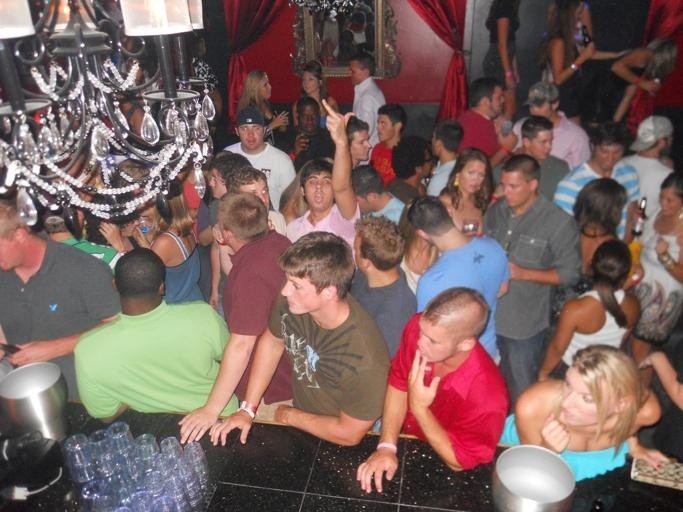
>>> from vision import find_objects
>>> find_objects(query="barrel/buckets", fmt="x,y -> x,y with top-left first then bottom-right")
1,362 -> 70,447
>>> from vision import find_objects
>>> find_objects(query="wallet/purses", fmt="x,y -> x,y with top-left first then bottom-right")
629,458 -> 682,491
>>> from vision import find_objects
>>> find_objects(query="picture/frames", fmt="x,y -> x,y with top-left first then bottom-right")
292,2 -> 403,77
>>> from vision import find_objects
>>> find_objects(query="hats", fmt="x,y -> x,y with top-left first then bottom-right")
520,81 -> 559,108
627,115 -> 673,152
237,106 -> 265,128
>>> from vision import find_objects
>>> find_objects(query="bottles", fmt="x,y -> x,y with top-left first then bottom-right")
581,23 -> 591,48
631,195 -> 647,237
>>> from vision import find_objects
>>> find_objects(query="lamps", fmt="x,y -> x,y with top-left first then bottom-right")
0,0 -> 229,245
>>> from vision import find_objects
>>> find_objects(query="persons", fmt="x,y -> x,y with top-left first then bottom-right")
529,0 -> 679,110
2,60 -> 683,498
482,1 -> 522,118
311,0 -> 377,68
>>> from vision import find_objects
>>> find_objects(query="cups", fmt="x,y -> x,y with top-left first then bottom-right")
460,219 -> 480,234
648,77 -> 660,97
61,421 -> 210,512
137,214 -> 155,236
